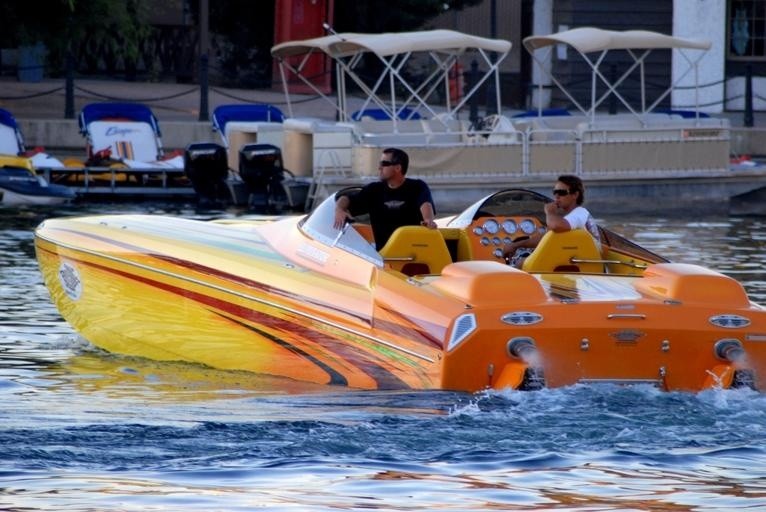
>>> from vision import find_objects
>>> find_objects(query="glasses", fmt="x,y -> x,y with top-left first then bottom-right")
553,189 -> 569,196
381,161 -> 395,166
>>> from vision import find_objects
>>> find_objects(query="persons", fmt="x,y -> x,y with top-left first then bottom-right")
333,148 -> 437,252
503,174 -> 609,274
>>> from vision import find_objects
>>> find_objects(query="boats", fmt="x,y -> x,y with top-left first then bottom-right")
36,185 -> 766,392
182,21 -> 764,209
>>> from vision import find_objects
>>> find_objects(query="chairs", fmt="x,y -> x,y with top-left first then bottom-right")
1,112 -> 64,188
377,224 -> 454,280
509,109 -> 572,117
73,102 -> 189,172
350,108 -> 423,121
211,102 -> 291,148
522,226 -> 607,277
659,110 -> 710,120
